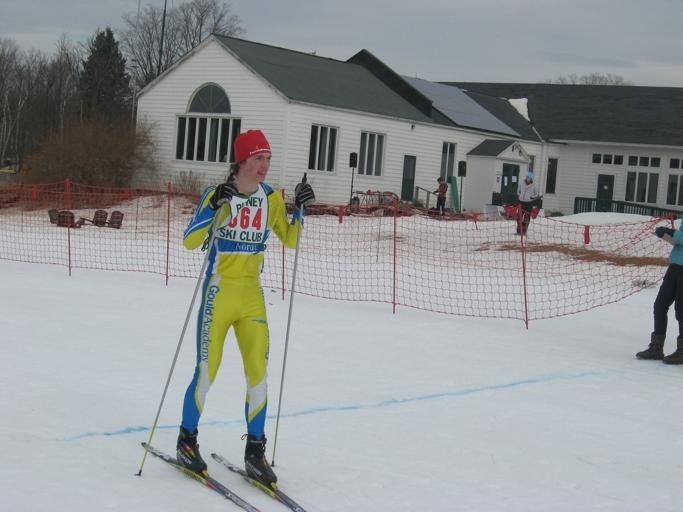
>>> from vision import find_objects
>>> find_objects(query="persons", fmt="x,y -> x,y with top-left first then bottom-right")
432,177 -> 448,217
176,129 -> 317,485
634,218 -> 682,364
513,172 -> 538,234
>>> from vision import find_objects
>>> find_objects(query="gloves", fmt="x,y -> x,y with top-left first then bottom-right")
210,184 -> 238,208
295,183 -> 315,209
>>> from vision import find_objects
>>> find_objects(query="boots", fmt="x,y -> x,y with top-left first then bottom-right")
663,336 -> 683,364
177,426 -> 207,471
637,332 -> 666,359
245,435 -> 277,485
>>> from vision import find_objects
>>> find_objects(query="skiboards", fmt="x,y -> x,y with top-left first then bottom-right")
141,442 -> 306,512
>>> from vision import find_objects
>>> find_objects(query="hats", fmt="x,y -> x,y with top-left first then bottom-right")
526,172 -> 533,179
234,131 -> 271,165
